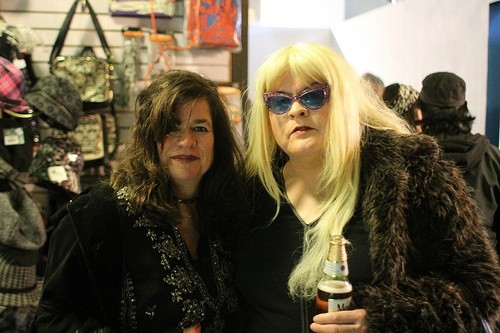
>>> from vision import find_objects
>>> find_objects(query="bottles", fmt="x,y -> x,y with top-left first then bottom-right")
315,235 -> 353,314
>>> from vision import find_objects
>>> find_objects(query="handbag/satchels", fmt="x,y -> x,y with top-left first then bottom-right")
48,0 -> 118,165
1,55 -> 28,112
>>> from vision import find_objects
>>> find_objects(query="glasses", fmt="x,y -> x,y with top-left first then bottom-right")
260,86 -> 330,115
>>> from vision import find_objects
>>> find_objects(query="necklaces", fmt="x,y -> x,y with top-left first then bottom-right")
174,195 -> 198,205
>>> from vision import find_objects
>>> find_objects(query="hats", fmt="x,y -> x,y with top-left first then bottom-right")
1,185 -> 48,251
31,128 -> 84,196
1,22 -> 36,54
24,75 -> 81,131
419,72 -> 466,108
1,258 -> 41,306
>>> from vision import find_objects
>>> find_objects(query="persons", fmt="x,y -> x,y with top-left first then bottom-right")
29,69 -> 288,333
361,72 -> 500,238
231,42 -> 500,333
